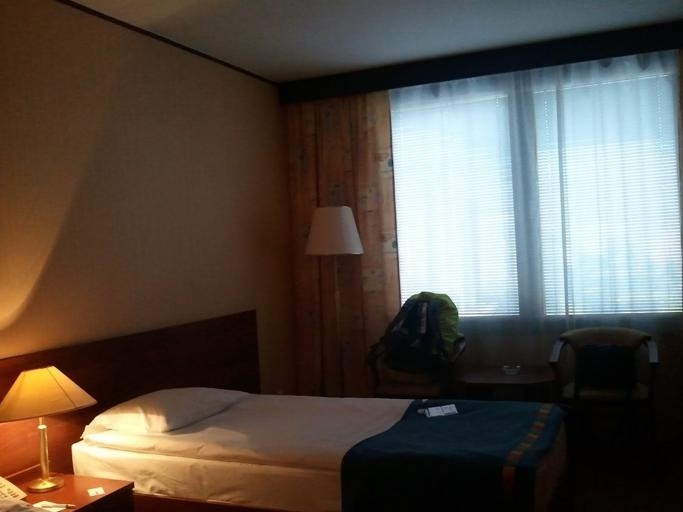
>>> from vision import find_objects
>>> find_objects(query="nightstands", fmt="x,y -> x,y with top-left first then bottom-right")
17,473 -> 134,512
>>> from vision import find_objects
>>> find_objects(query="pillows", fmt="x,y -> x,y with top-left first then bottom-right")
88,387 -> 248,432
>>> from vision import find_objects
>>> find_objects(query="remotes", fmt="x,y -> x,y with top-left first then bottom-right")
426,404 -> 459,418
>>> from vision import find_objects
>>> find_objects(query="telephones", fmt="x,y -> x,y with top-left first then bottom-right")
0,476 -> 28,505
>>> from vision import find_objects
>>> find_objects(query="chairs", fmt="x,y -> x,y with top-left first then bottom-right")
367,292 -> 458,398
548,327 -> 659,440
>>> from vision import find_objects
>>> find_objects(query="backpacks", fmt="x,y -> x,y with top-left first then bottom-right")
377,292 -> 459,386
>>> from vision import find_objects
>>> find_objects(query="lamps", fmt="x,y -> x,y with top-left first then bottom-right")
304,206 -> 364,397
0,366 -> 97,492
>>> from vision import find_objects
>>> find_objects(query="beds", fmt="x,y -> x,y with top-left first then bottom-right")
0,310 -> 569,512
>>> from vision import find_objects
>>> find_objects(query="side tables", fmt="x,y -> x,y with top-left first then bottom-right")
456,368 -> 557,387
